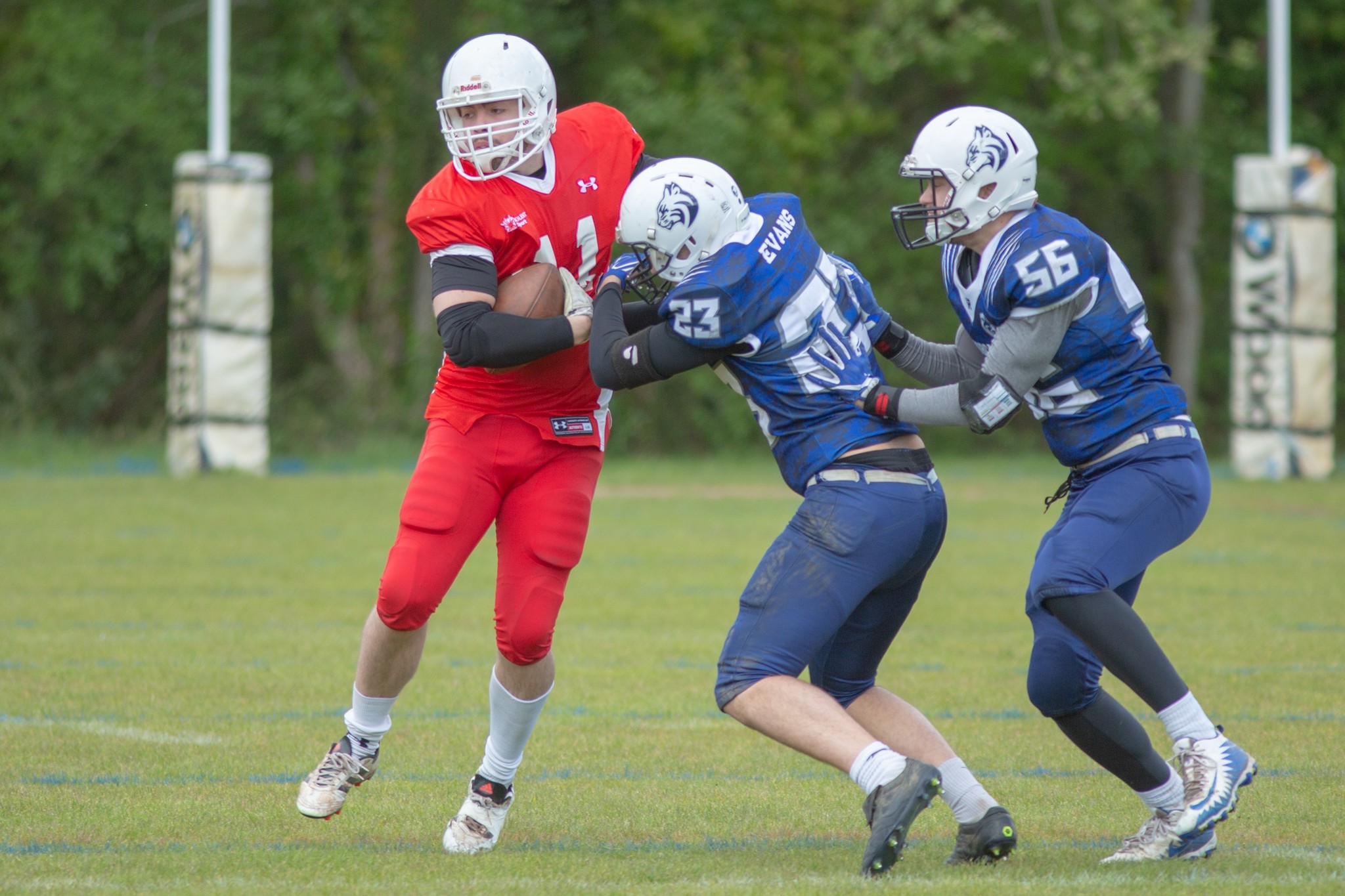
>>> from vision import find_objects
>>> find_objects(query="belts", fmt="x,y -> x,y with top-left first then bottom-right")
806,468 -> 938,485
1076,424 -> 1200,469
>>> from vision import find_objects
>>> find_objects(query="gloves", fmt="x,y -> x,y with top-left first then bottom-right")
560,266 -> 595,345
827,253 -> 892,344
805,322 -> 880,405
595,253 -> 648,293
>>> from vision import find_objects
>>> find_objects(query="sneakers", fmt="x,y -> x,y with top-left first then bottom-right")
946,806 -> 1018,865
442,774 -> 515,856
296,735 -> 380,821
861,755 -> 944,877
1166,724 -> 1259,842
1100,808 -> 1217,866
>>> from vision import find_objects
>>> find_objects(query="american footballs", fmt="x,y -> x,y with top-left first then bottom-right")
481,263 -> 565,374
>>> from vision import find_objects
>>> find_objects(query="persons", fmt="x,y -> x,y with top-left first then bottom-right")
293,34 -> 646,856
824,105 -> 1260,868
589,155 -> 1022,879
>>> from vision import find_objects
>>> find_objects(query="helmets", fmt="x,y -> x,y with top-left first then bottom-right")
442,33 -> 558,145
898,105 -> 1037,246
616,156 -> 749,282
